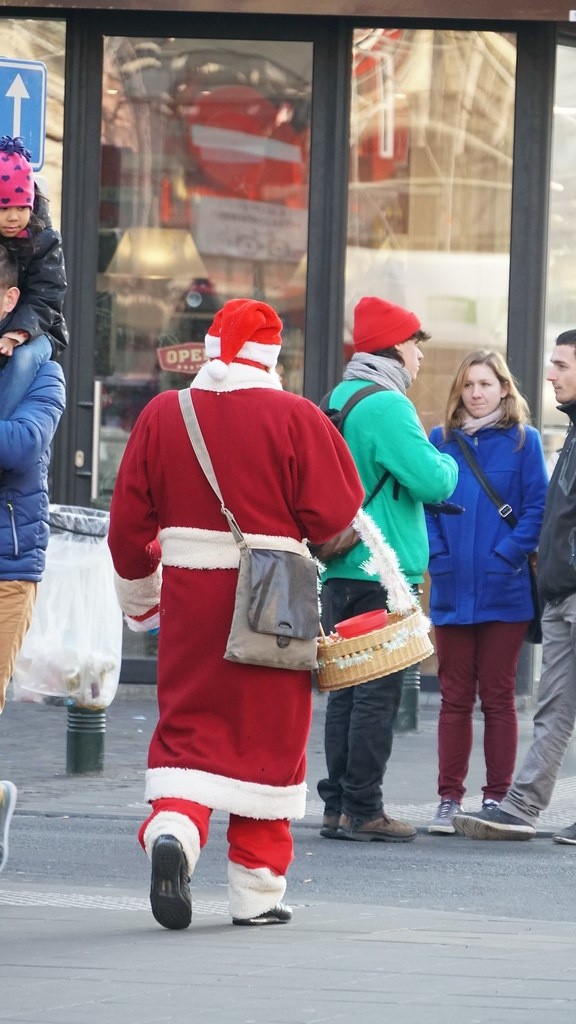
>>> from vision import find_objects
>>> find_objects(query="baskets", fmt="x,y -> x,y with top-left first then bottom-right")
311,510 -> 434,690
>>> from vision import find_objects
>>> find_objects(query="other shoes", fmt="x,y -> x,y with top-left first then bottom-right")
0,780 -> 18,872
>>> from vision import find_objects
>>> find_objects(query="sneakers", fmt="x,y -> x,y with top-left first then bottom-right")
320,812 -> 343,838
233,901 -> 291,925
483,803 -> 500,812
150,835 -> 192,929
427,800 -> 458,834
337,810 -> 418,843
553,823 -> 576,846
451,806 -> 537,840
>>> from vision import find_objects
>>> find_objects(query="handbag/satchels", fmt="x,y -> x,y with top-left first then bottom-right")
526,552 -> 546,645
225,550 -> 319,672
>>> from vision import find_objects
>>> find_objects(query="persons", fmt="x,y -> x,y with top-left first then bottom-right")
450,329 -> 576,845
425,349 -> 549,835
0,134 -> 68,869
317,298 -> 460,843
108,298 -> 362,931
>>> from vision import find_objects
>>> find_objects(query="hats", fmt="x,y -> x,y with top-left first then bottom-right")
0,134 -> 35,208
174,280 -> 215,312
352,297 -> 421,353
205,298 -> 284,381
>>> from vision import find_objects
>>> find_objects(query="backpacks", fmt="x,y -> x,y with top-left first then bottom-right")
309,383 -> 392,561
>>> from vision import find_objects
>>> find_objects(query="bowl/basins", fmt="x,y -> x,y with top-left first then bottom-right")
334,609 -> 387,640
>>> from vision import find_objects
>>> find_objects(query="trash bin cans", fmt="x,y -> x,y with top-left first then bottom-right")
12,503 -> 125,711
